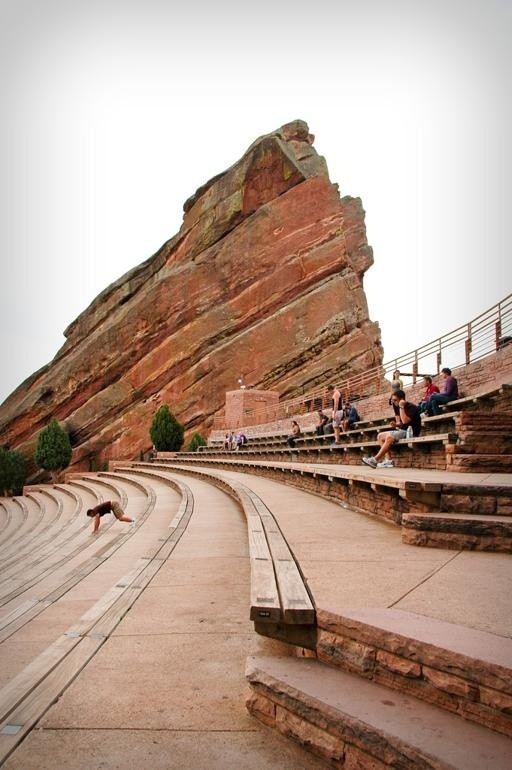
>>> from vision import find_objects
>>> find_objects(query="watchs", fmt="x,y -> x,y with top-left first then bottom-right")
399,406 -> 404,408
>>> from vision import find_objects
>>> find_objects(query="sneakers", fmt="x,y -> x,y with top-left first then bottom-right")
377,458 -> 394,468
361,456 -> 378,469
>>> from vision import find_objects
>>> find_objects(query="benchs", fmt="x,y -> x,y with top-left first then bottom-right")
2,384 -> 511,757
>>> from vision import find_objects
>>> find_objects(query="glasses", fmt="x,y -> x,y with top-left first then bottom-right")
391,399 -> 397,402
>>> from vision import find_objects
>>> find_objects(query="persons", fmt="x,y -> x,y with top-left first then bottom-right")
328,384 -> 344,446
86,500 -> 136,536
286,420 -> 300,448
388,369 -> 404,423
219,431 -> 248,451
420,367 -> 458,418
361,389 -> 422,469
416,376 -> 440,414
314,402 -> 359,443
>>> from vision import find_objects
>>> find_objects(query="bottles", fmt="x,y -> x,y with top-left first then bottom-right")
406,425 -> 413,439
